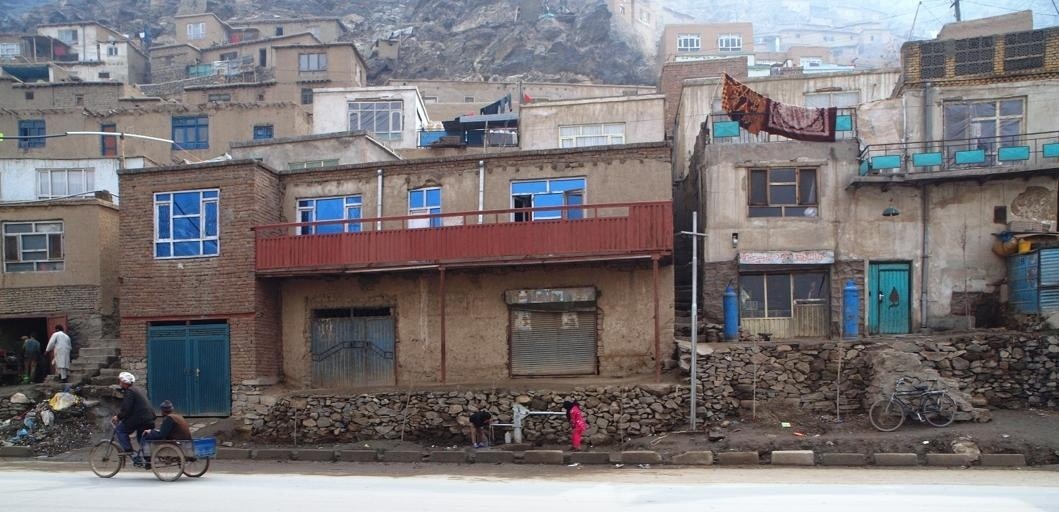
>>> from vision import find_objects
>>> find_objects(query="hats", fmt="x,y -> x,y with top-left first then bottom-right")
160,400 -> 174,411
119,371 -> 135,384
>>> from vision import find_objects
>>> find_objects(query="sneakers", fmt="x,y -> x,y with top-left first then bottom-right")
117,452 -> 133,456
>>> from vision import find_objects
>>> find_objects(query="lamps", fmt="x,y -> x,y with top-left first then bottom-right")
882,190 -> 900,217
732,233 -> 738,249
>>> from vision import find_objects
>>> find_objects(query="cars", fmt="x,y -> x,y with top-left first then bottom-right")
0,347 -> 18,381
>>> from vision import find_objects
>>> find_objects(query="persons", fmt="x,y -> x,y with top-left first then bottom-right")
142,399 -> 192,440
21,333 -> 42,384
112,371 -> 157,470
469,411 -> 498,449
15,336 -> 29,384
564,400 -> 587,452
43,324 -> 72,383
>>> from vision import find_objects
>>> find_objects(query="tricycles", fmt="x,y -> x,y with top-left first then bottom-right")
87,413 -> 219,484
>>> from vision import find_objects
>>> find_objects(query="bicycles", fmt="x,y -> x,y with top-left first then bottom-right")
868,372 -> 958,432
